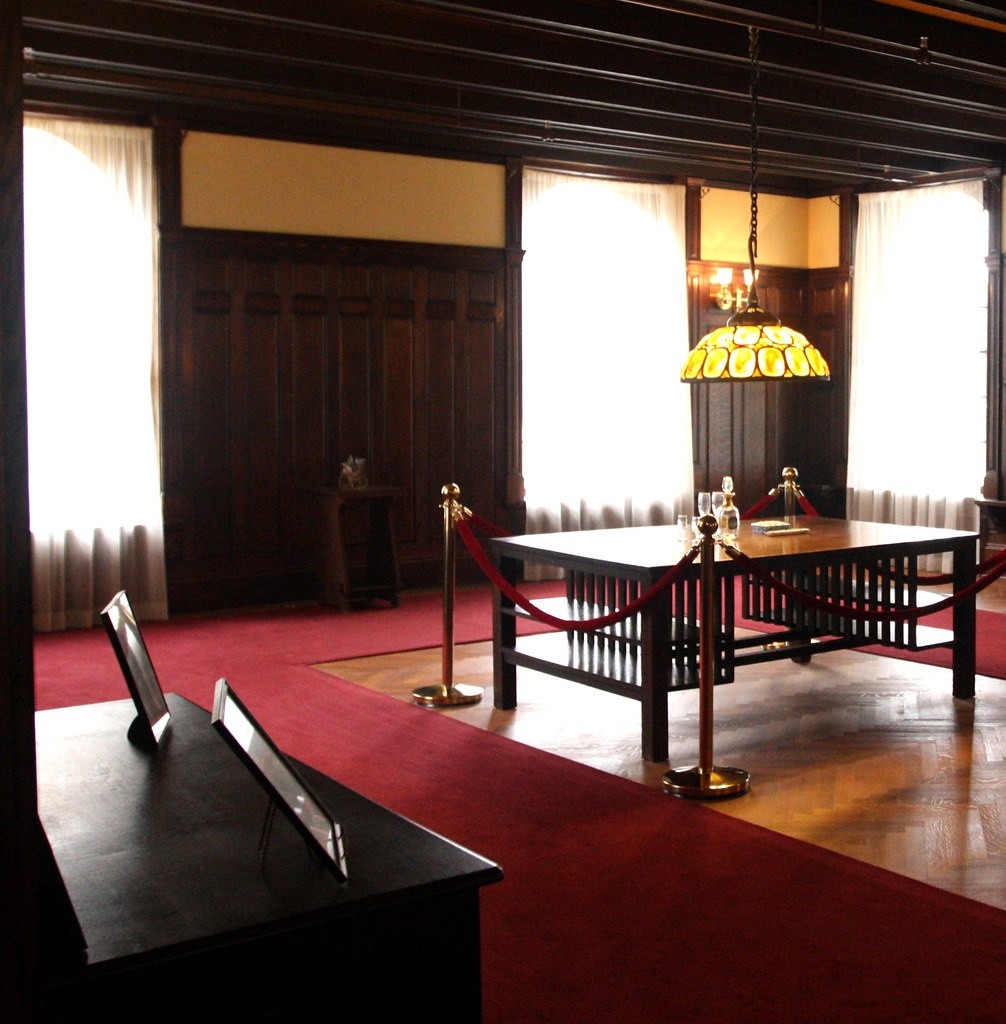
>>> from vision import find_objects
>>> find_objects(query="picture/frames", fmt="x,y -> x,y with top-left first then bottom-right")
101,587 -> 173,749
211,676 -> 349,882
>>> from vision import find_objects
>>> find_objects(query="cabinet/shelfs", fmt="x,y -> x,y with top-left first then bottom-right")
309,483 -> 404,614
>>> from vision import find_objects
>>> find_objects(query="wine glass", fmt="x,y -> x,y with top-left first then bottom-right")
677,515 -> 689,542
712,491 -> 727,538
692,517 -> 702,543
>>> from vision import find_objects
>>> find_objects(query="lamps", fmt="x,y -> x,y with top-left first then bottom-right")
705,266 -> 762,313
681,26 -> 832,385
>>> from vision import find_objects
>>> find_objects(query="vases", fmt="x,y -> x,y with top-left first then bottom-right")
338,471 -> 368,489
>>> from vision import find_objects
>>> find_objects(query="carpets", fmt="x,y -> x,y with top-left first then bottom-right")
35,566 -> 1006,1024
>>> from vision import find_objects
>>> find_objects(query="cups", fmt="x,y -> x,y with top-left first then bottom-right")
698,493 -> 711,518
722,476 -> 735,494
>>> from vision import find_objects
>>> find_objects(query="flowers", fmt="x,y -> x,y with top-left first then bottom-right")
341,456 -> 366,479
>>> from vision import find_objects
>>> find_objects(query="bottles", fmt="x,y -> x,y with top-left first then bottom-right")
718,492 -> 741,540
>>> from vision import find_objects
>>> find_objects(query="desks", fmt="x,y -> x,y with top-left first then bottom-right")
487,511 -> 986,765
35,698 -> 508,1023
